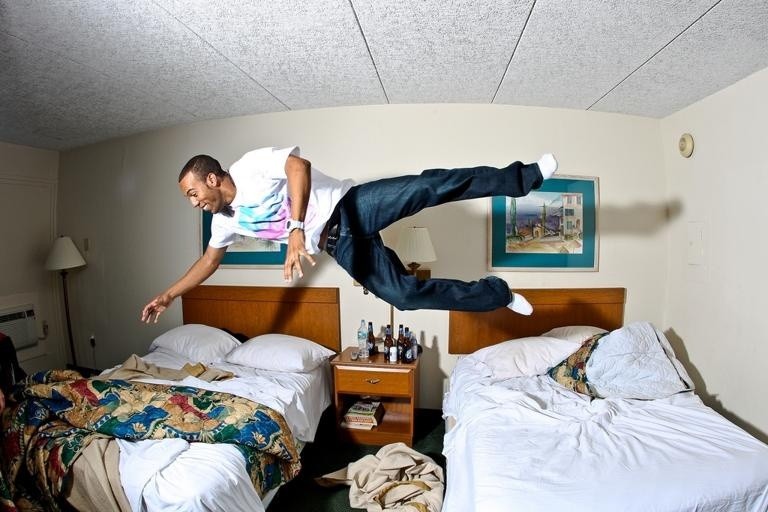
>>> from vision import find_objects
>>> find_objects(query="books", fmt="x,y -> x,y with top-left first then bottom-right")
340,402 -> 384,431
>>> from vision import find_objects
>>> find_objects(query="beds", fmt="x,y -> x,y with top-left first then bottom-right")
0,286 -> 340,512
442,285 -> 766,510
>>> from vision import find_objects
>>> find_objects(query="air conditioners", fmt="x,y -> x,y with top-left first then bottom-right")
0,303 -> 39,351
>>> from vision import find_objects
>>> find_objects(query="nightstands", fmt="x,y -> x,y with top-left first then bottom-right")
329,346 -> 418,451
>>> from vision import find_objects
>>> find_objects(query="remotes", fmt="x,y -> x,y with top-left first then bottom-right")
351,351 -> 358,361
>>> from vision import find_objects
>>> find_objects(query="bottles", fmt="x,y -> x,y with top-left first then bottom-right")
357,319 -> 370,359
383,324 -> 418,363
366,321 -> 375,357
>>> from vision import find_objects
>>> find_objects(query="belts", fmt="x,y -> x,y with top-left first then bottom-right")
326,208 -> 340,258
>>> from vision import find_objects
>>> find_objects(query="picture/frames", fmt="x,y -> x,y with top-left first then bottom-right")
199,202 -> 300,270
486,173 -> 601,273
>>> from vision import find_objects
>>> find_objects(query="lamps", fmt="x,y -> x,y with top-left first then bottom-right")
41,234 -> 90,369
393,227 -> 438,280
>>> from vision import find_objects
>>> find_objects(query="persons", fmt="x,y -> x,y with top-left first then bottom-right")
140,144 -> 558,325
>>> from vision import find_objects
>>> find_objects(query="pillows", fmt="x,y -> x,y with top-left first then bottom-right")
541,326 -> 609,346
147,321 -> 242,366
224,328 -> 336,374
461,331 -> 582,381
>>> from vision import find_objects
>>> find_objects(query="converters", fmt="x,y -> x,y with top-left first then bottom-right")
90,339 -> 95,347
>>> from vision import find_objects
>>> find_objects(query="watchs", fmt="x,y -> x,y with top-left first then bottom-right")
285,218 -> 305,233
